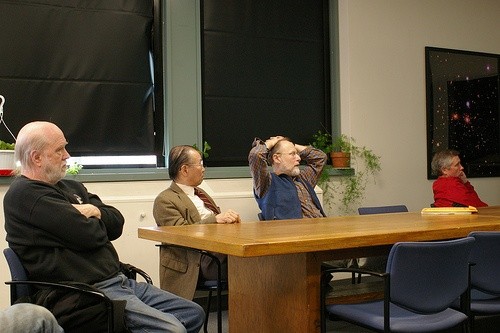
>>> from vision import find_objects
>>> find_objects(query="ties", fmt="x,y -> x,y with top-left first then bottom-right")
194,188 -> 220,214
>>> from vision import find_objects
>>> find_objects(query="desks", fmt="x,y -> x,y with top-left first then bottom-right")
138,206 -> 500,333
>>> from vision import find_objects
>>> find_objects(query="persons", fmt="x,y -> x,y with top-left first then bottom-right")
248,136 -> 372,299
153,145 -> 241,301
432,151 -> 489,207
4,120 -> 206,333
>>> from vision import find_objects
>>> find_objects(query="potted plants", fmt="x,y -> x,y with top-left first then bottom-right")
0,139 -> 16,176
307,130 -> 383,215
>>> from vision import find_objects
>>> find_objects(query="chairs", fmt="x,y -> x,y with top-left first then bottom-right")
155,245 -> 229,333
351,205 -> 408,284
3,248 -> 153,333
319,232 -> 500,333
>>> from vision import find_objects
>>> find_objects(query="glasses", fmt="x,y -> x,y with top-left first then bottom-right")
180,160 -> 203,171
275,152 -> 300,156
